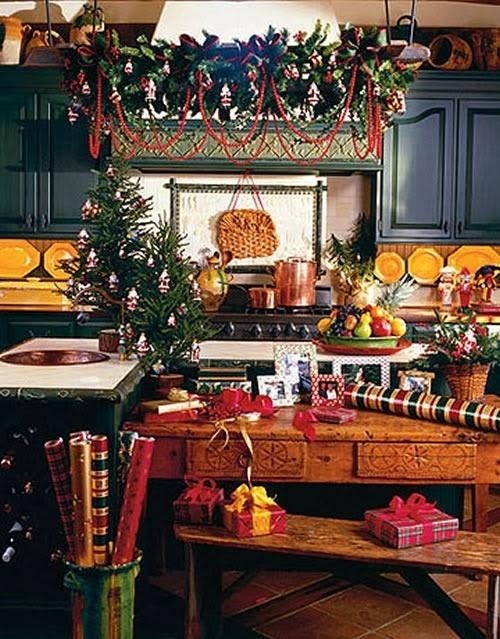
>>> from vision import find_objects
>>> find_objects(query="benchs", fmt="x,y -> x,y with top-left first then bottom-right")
173,513 -> 500,639
0,337 -> 145,492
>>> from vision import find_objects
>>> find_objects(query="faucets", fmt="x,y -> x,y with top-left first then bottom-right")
70,288 -> 126,328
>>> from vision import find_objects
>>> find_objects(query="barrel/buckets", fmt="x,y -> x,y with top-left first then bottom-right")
273,257 -> 317,307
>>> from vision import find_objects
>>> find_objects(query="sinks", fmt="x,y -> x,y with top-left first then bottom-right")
0,348 -> 109,366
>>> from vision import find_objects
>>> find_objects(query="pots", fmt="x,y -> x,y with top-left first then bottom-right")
217,256 -> 318,308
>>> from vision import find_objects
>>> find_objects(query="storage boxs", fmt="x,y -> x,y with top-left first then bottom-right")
363,493 -> 460,549
221,484 -> 286,539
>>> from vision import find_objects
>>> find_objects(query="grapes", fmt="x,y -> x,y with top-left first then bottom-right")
330,304 -> 360,338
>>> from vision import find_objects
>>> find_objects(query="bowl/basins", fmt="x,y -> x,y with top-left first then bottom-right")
326,333 -> 402,348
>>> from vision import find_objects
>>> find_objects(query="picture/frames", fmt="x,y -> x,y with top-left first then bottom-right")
257,345 -> 432,407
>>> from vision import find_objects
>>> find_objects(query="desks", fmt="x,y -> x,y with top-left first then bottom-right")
124,394 -> 500,580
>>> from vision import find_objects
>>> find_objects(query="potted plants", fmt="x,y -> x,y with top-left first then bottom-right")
52,148 -> 155,353
119,211 -> 211,398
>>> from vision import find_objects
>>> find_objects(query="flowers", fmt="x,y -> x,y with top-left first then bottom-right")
419,307 -> 500,371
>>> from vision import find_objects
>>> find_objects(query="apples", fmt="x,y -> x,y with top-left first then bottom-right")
343,304 -> 406,338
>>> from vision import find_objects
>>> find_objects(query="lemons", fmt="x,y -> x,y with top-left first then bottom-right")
317,318 -> 330,334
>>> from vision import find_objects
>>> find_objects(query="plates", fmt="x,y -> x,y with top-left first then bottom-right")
0,238 -> 81,280
373,244 -> 500,286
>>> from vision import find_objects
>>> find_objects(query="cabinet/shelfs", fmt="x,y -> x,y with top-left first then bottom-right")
372,71 -> 500,244
0,65 -> 111,240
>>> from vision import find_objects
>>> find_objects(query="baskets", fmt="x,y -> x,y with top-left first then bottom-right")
443,363 -> 492,404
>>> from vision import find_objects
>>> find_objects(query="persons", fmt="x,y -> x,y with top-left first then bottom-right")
264,350 -> 428,401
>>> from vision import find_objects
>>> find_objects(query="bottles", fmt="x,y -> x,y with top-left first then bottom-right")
196,255 -> 231,311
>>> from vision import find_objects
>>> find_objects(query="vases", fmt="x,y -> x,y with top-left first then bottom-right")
197,270 -> 229,312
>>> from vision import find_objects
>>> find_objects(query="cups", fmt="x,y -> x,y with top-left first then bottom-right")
98,329 -> 121,353
428,34 -> 473,71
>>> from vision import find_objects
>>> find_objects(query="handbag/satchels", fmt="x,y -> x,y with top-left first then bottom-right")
216,171 -> 281,259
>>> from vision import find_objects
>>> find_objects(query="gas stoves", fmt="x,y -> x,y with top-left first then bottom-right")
195,306 -> 330,340
